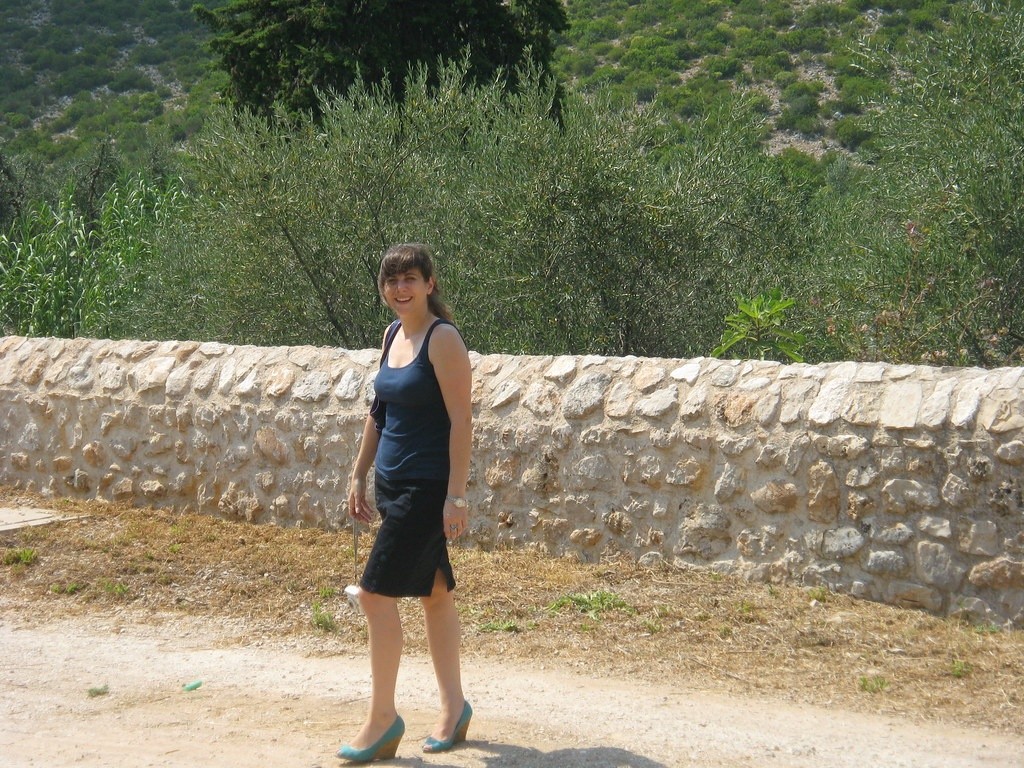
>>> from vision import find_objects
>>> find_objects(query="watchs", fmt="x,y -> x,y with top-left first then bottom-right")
447,496 -> 466,508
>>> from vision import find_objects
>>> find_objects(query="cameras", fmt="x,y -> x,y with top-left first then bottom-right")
345,585 -> 363,608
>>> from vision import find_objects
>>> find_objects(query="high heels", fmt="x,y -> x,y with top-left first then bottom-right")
422,701 -> 472,753
335,715 -> 405,762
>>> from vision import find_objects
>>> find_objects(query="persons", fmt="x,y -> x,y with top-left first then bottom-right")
334,243 -> 474,763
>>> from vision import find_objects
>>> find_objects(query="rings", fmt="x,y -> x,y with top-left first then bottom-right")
450,526 -> 456,531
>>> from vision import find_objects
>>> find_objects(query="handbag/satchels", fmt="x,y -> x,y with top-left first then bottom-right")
370,395 -> 387,439
